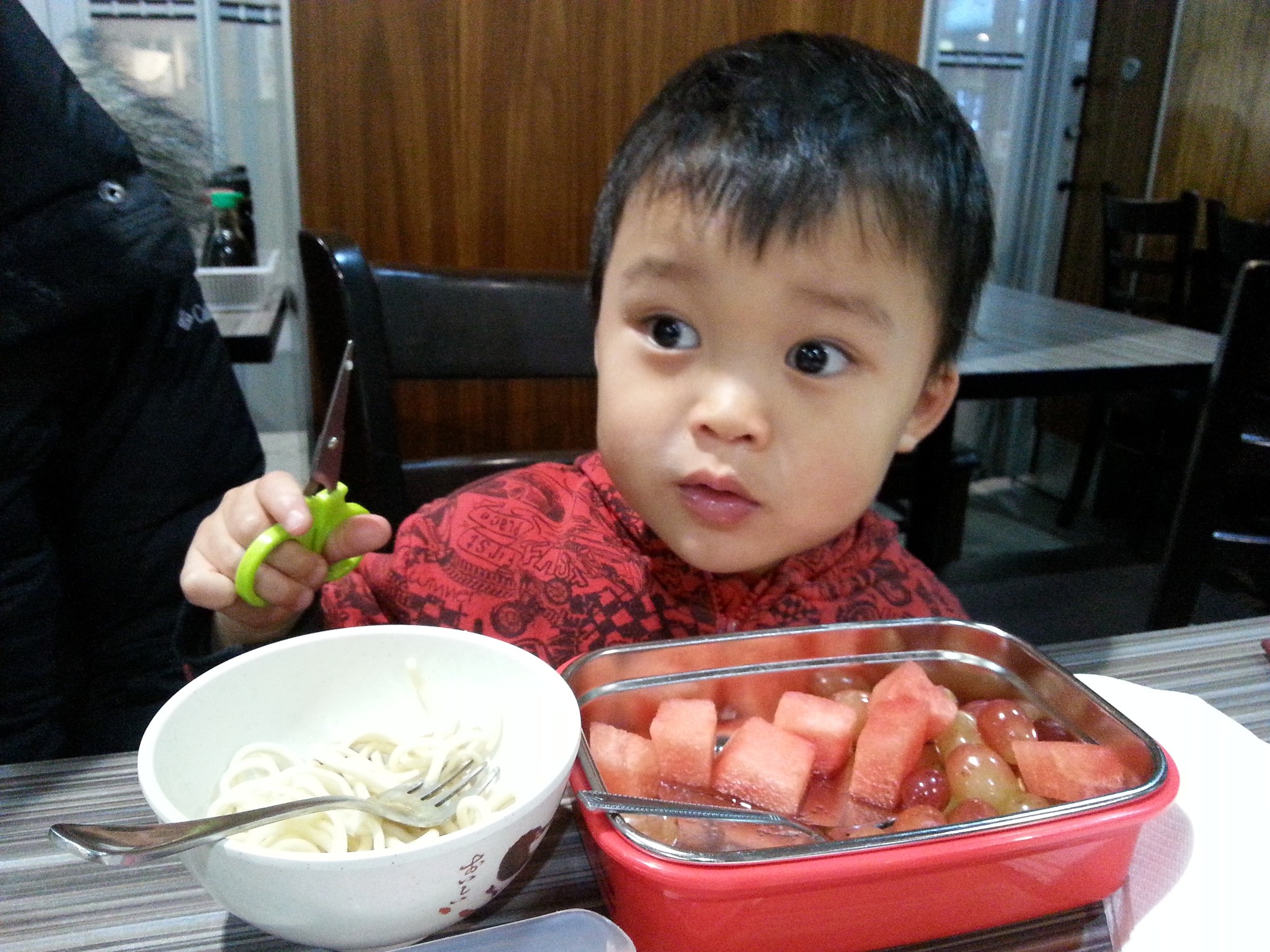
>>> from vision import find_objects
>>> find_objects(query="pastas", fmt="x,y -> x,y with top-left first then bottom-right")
208,735 -> 517,853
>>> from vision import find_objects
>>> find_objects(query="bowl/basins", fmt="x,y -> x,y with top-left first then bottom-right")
138,624 -> 581,952
557,617 -> 1181,951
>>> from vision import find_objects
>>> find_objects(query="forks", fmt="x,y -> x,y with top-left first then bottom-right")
578,789 -> 900,844
48,753 -> 501,867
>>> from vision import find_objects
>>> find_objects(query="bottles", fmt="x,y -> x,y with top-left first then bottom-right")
199,164 -> 259,268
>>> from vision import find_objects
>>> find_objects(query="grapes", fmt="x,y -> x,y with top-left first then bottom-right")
834,688 -> 1075,837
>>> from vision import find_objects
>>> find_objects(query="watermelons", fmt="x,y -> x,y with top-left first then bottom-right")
589,660 -> 957,851
1011,740 -> 1141,802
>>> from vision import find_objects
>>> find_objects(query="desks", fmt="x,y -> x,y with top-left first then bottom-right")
0,618 -> 1270,952
877,283 -> 1226,583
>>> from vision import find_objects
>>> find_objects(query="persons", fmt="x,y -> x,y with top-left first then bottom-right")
0,0 -> 272,762
179,31 -> 999,670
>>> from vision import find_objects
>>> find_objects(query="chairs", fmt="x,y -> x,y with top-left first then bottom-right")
297,229 -> 592,555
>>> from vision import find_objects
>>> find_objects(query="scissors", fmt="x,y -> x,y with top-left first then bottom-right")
232,338 -> 373,608
